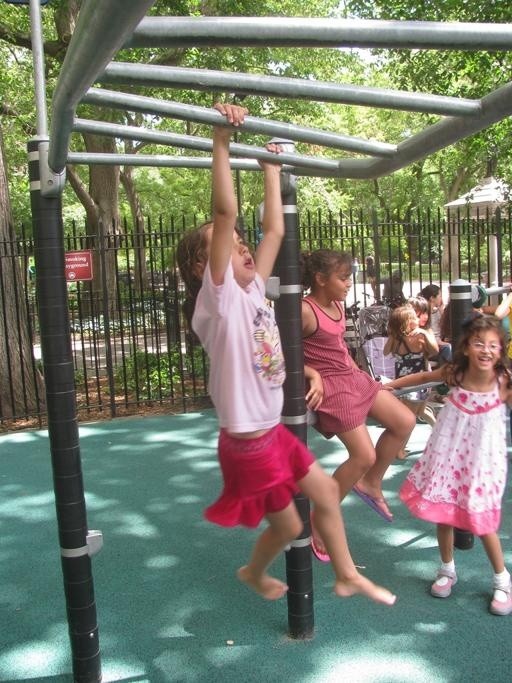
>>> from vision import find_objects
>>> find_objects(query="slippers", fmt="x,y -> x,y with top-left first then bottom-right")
309,511 -> 331,562
351,483 -> 395,523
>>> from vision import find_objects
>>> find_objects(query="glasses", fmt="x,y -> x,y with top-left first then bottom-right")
467,342 -> 503,351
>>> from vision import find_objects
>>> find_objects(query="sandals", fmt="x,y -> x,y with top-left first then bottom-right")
490,578 -> 512,616
430,570 -> 458,598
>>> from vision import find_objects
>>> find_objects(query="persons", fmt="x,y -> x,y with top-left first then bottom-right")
382,283 -> 511,417
299,246 -> 417,566
379,314 -> 512,618
366,252 -> 374,282
379,263 -> 406,299
177,102 -> 401,608
351,256 -> 360,283
